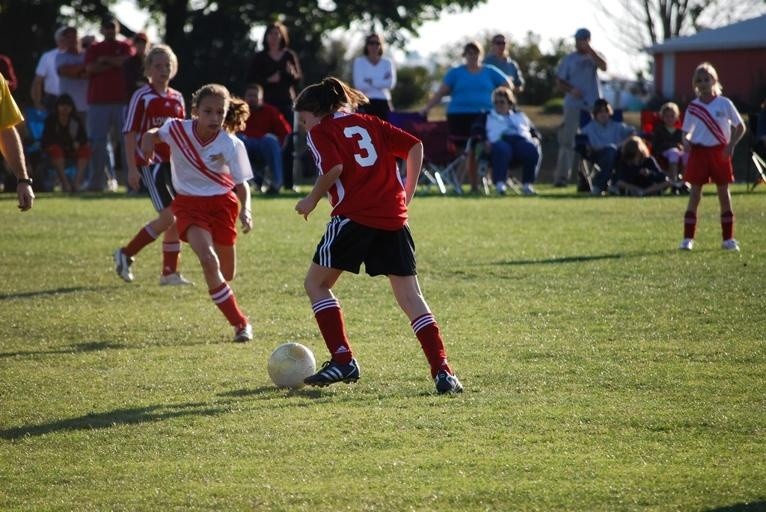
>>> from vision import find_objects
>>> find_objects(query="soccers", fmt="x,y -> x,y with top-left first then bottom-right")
269,344 -> 315,390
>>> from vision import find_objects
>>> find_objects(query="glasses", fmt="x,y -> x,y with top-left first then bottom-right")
493,42 -> 505,46
367,40 -> 380,46
494,100 -> 507,105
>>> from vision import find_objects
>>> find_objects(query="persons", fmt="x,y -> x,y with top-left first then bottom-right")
141,83 -> 255,341
114,44 -> 194,286
0,72 -> 35,212
680,62 -> 747,251
292,76 -> 463,394
0,54 -> 18,194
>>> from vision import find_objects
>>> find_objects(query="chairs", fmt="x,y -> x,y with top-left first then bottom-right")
388,111 -> 523,196
575,109 -> 623,191
641,110 -> 692,191
747,110 -> 766,191
27,108 -> 77,192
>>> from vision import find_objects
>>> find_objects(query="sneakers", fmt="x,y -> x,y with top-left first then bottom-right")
721,238 -> 738,251
551,177 -> 692,195
158,273 -> 195,288
433,370 -> 463,394
681,238 -> 693,251
111,246 -> 135,284
303,358 -> 361,387
233,323 -> 254,341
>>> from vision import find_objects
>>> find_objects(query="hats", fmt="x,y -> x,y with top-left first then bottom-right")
573,28 -> 591,39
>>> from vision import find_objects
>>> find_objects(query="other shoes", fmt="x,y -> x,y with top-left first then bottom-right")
254,178 -> 298,198
54,178 -> 117,193
451,179 -> 537,198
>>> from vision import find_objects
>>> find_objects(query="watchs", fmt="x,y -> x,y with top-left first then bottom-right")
16,178 -> 33,185
